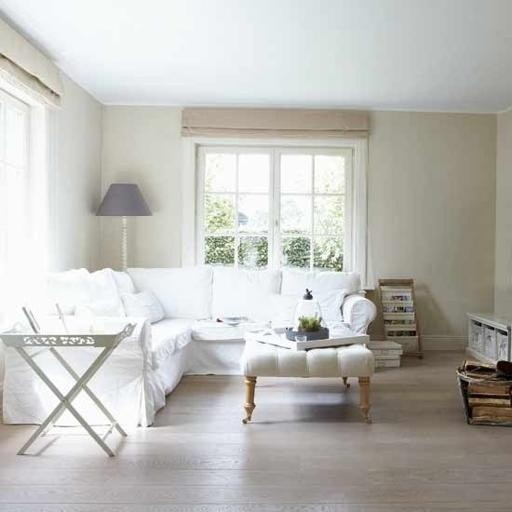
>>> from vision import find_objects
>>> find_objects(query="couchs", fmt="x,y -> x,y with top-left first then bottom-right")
2,265 -> 377,428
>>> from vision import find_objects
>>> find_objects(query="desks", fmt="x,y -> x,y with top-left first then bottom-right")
1,322 -> 137,457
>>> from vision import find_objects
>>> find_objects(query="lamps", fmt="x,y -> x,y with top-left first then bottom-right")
96,184 -> 153,271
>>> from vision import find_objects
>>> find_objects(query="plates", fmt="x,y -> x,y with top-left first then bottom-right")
258,325 -> 369,350
285,327 -> 329,340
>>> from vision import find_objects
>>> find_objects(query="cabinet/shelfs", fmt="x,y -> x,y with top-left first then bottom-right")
464,311 -> 512,365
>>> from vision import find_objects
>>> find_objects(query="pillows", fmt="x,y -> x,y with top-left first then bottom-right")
77,299 -> 125,319
121,290 -> 166,325
267,289 -> 345,328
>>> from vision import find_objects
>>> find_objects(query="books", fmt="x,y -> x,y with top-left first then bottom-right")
368,340 -> 403,368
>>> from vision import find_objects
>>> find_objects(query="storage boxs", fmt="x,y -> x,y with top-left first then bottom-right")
456,361 -> 512,427
